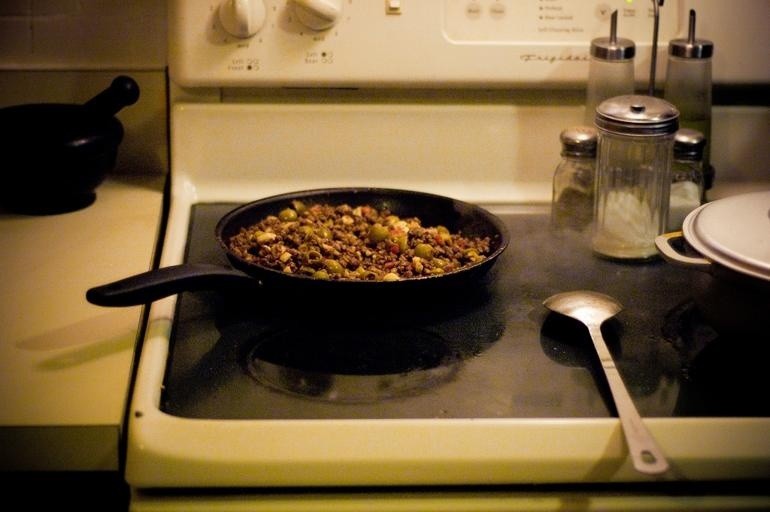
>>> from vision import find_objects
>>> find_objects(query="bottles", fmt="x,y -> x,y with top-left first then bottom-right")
593,93 -> 680,260
663,11 -> 715,186
667,126 -> 706,234
583,8 -> 636,128
552,126 -> 598,228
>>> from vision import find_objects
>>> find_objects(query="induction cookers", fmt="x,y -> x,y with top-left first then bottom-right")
159,203 -> 768,415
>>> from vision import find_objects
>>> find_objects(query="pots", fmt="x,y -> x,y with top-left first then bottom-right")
87,187 -> 511,327
656,193 -> 766,320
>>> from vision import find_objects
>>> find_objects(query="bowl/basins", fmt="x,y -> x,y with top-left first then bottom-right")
0,100 -> 123,216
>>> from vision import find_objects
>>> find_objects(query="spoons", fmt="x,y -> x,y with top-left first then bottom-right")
540,290 -> 669,476
